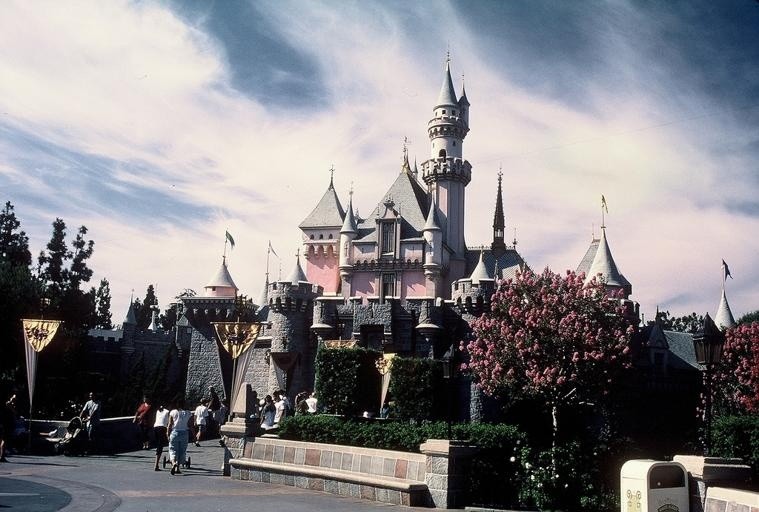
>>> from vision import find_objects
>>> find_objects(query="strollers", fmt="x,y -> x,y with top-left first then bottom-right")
163,427 -> 190,468
54,416 -> 90,457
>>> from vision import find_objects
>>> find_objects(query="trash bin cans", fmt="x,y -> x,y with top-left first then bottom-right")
620,459 -> 689,512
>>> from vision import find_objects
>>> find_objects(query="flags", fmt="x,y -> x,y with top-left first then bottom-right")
226,232 -> 235,249
602,195 -> 609,214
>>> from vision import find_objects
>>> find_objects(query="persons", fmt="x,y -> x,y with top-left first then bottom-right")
1,389 -> 317,474
362,400 -> 396,420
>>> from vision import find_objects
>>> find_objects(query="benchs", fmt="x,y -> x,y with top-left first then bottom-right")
228,456 -> 428,506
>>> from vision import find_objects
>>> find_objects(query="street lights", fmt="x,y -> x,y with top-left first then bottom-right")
693,311 -> 726,456
